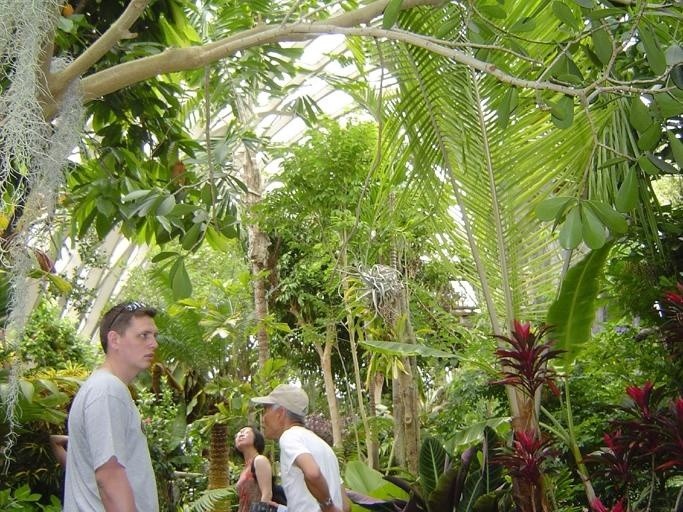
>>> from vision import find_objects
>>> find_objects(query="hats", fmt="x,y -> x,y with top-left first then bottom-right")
250,383 -> 310,417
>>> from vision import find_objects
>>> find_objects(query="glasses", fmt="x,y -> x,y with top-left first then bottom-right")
107,300 -> 149,333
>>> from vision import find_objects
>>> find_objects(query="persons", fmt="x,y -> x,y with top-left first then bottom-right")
47,434 -> 68,469
62,298 -> 162,511
250,382 -> 354,512
262,496 -> 287,512
233,424 -> 274,512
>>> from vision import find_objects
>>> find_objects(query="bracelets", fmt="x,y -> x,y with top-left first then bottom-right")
320,497 -> 333,510
